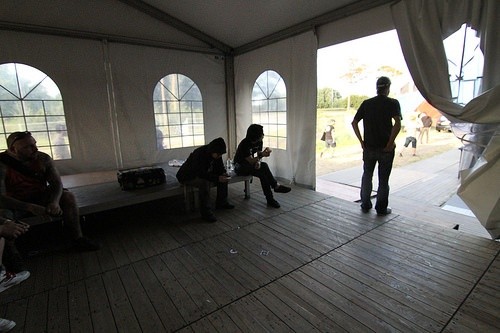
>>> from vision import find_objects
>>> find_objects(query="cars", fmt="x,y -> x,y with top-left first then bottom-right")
436,115 -> 452,132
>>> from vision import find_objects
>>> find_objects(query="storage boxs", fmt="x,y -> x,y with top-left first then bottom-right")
116,165 -> 166,191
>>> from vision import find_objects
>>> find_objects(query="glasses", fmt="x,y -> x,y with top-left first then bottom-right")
10,130 -> 31,146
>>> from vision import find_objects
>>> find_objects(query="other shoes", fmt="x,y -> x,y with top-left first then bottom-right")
70,236 -> 100,250
5,249 -> 26,272
377,208 -> 392,216
361,207 -> 369,213
274,185 -> 292,193
266,198 -> 281,208
216,203 -> 235,209
200,209 -> 217,223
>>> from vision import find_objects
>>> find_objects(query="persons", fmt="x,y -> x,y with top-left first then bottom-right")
176,137 -> 235,223
319,119 -> 337,159
0,131 -> 90,275
155,127 -> 164,151
352,76 -> 403,217
54,123 -> 72,160
233,123 -> 292,208
0,193 -> 64,332
398,110 -> 453,158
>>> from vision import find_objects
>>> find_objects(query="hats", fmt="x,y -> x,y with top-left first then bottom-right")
376,76 -> 391,94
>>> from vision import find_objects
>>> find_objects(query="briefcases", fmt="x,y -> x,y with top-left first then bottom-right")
117,165 -> 166,191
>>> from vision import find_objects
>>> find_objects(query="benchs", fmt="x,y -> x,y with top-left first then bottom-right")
21,164 -> 252,228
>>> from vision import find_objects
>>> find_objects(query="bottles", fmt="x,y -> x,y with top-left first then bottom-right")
226,158 -> 232,172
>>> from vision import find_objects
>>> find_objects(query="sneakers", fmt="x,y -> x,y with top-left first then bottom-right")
0,318 -> 16,333
0,266 -> 31,293
